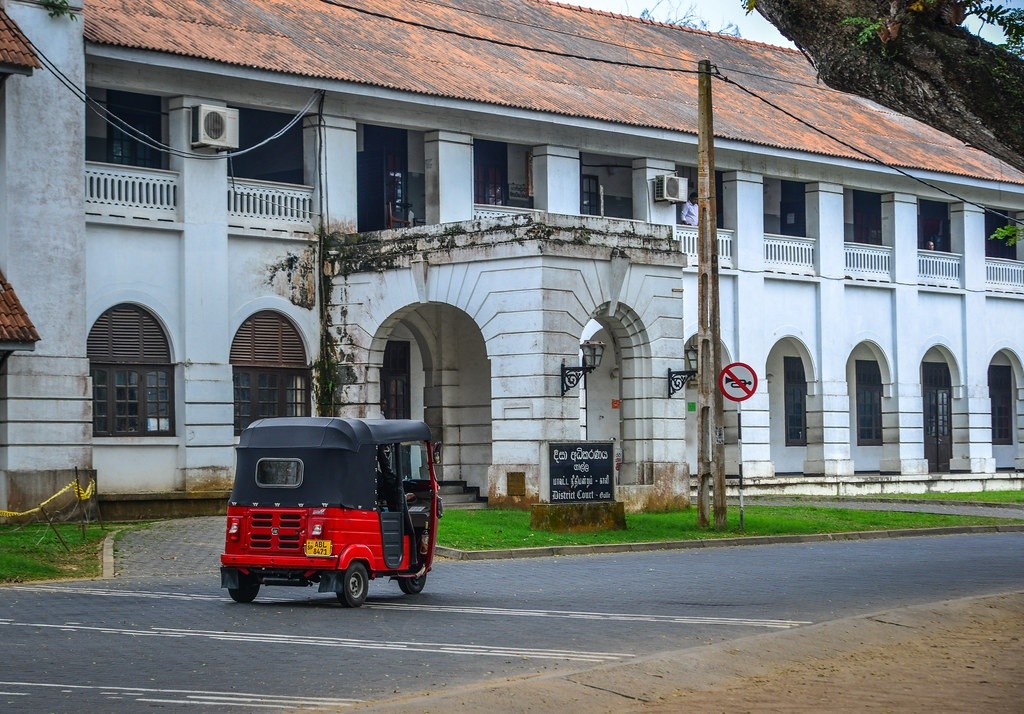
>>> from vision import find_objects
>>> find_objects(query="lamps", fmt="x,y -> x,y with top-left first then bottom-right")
666,345 -> 699,399
560,339 -> 606,398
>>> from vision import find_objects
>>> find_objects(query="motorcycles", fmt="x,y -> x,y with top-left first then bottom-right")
219,418 -> 446,608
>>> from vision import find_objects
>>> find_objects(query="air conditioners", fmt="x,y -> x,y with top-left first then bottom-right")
654,174 -> 688,205
189,103 -> 239,154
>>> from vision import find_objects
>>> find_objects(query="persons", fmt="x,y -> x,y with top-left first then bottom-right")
927,240 -> 934,251
681,193 -> 698,226
406,492 -> 414,500
381,399 -> 386,421
